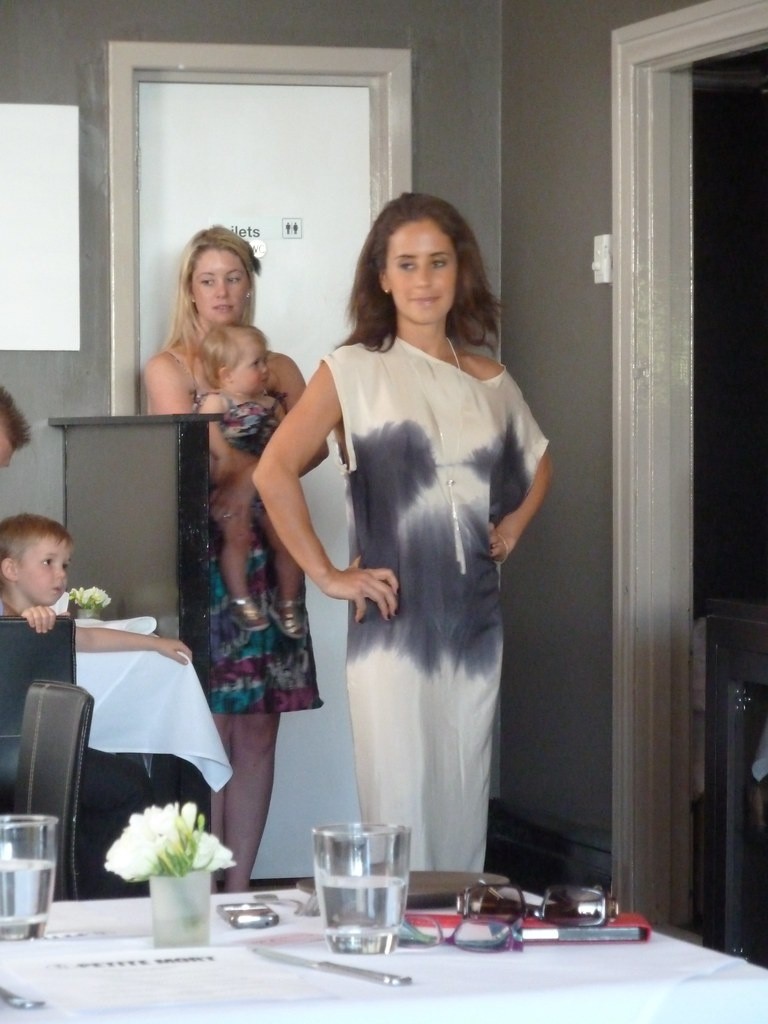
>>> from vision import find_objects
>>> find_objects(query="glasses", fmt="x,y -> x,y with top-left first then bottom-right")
457,879 -> 620,927
401,913 -> 524,954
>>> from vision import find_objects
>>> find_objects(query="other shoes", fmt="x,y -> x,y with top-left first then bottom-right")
226,596 -> 271,632
267,599 -> 309,639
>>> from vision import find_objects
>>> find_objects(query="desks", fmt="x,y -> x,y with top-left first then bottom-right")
0,888 -> 768,1024
74,616 -> 234,900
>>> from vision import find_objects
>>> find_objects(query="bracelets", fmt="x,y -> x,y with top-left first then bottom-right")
491,529 -> 509,565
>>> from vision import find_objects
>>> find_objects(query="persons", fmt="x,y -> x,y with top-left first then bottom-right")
0,382 -> 194,666
250,191 -> 552,874
143,225 -> 324,895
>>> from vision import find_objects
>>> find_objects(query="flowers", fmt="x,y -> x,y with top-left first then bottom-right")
69,586 -> 112,616
104,801 -> 237,882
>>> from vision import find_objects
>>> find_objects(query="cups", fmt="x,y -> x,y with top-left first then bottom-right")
149,871 -> 211,948
0,816 -> 59,940
78,608 -> 101,621
315,824 -> 411,953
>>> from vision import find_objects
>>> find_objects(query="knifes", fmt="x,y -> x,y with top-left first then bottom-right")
253,949 -> 413,986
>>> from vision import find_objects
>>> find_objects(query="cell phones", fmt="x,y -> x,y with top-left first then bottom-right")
217,902 -> 279,930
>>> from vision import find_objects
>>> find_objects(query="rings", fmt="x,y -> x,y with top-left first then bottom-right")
223,513 -> 232,519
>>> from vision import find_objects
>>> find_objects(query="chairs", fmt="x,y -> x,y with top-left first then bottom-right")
0,615 -> 76,815
14,679 -> 95,900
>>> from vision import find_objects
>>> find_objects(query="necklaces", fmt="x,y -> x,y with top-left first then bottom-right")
391,336 -> 467,576
